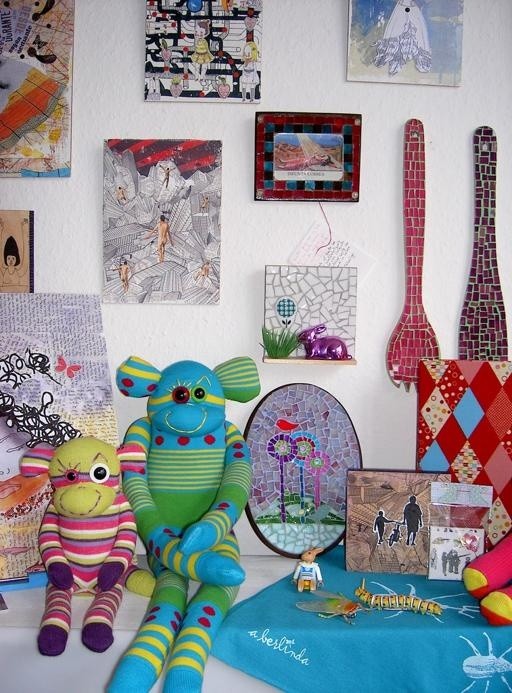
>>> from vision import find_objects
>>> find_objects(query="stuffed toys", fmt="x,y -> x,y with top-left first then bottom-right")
462,530 -> 512,626
38,357 -> 260,693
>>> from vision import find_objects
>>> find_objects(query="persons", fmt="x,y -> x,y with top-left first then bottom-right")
373,498 -> 424,546
115,185 -> 127,202
199,193 -> 209,213
145,215 -> 172,262
277,155 -> 334,173
0,218 -> 31,286
442,549 -> 464,576
201,261 -> 210,278
160,166 -> 174,187
112,261 -> 131,291
